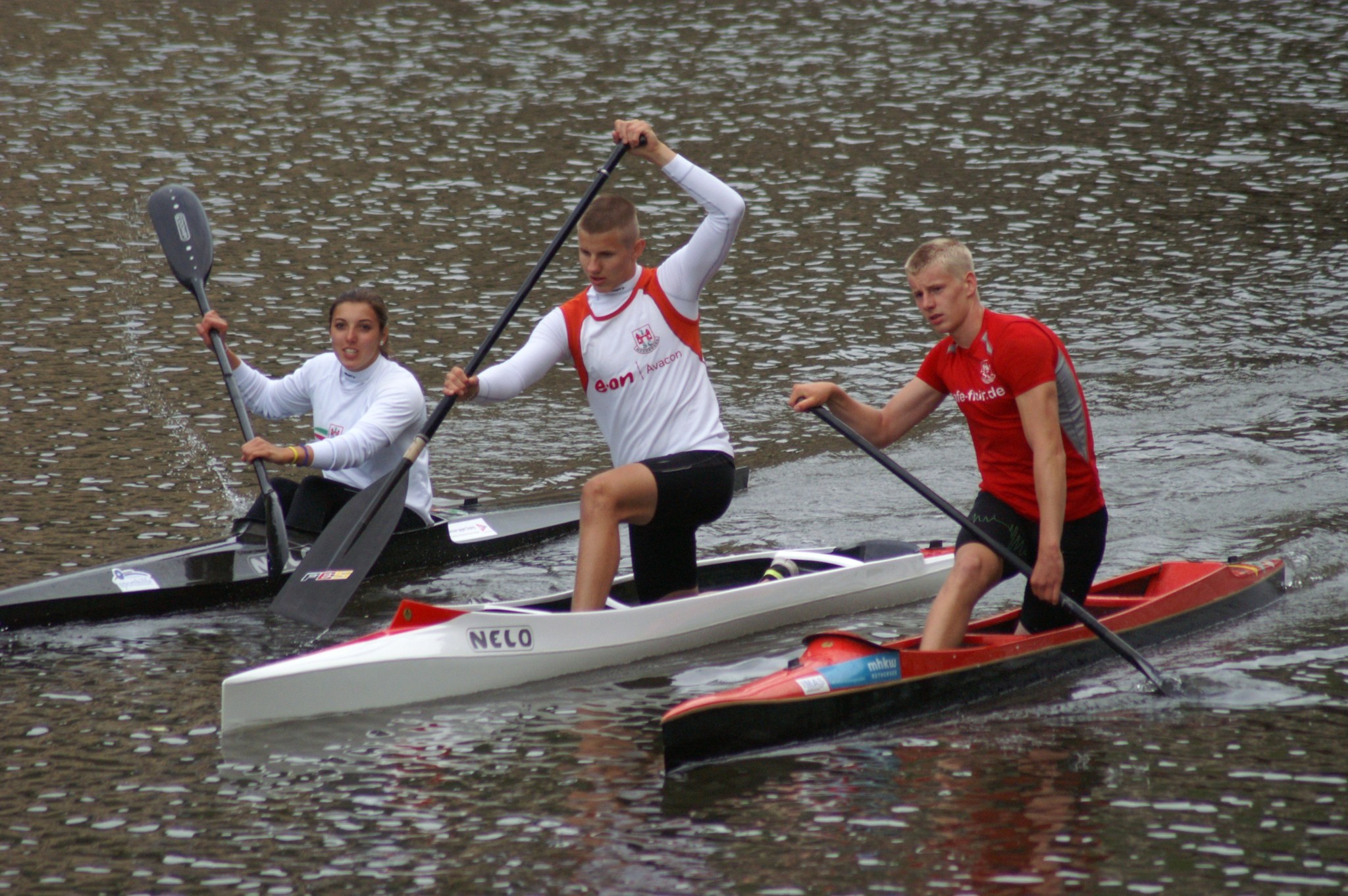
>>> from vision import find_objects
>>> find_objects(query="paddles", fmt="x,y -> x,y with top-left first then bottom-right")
795,396 -> 1193,702
144,182 -> 294,583
267,131 -> 648,633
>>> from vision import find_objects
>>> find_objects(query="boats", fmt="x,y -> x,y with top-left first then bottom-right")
220,540 -> 962,734
661,557 -> 1285,775
0,465 -> 749,630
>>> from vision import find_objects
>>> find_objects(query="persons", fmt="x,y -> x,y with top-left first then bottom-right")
789,239 -> 1109,650
442,120 -> 799,612
196,289 -> 434,532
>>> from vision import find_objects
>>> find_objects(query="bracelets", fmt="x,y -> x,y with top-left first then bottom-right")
288,446 -> 298,465
223,343 -> 228,352
296,445 -> 308,467
